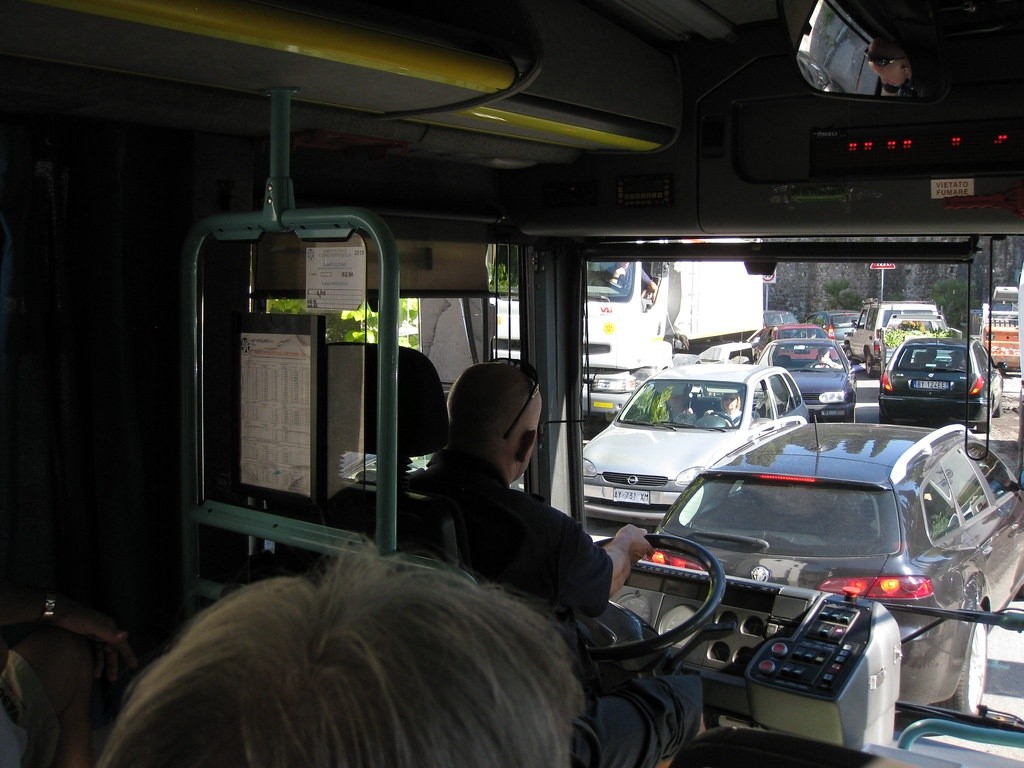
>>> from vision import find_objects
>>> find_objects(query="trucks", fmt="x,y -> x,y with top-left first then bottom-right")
492,238 -> 764,423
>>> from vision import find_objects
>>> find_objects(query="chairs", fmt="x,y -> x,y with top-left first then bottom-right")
325,340 -> 476,584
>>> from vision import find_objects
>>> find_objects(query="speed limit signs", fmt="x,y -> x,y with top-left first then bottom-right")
763,275 -> 774,280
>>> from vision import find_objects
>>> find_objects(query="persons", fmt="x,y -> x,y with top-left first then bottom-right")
810,347 -> 839,368
704,392 -> 755,426
670,393 -> 693,423
0,591 -> 139,768
92,564 -> 584,768
413,358 -> 702,768
864,38 -> 941,98
604,261 -> 656,299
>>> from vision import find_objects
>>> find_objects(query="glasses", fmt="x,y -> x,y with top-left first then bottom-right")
721,397 -> 735,401
486,358 -> 540,440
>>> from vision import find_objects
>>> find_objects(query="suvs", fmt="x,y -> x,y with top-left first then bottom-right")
842,299 -> 942,376
644,414 -> 1024,717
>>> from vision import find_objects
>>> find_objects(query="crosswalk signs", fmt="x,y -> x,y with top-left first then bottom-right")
869,263 -> 895,270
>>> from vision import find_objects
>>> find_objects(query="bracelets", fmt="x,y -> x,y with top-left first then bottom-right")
40,591 -> 56,622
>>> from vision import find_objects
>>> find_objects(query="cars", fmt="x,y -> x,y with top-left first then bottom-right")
750,338 -> 866,423
763,310 -> 802,343
880,336 -> 1007,433
794,311 -> 866,345
584,362 -> 809,527
755,324 -> 842,362
